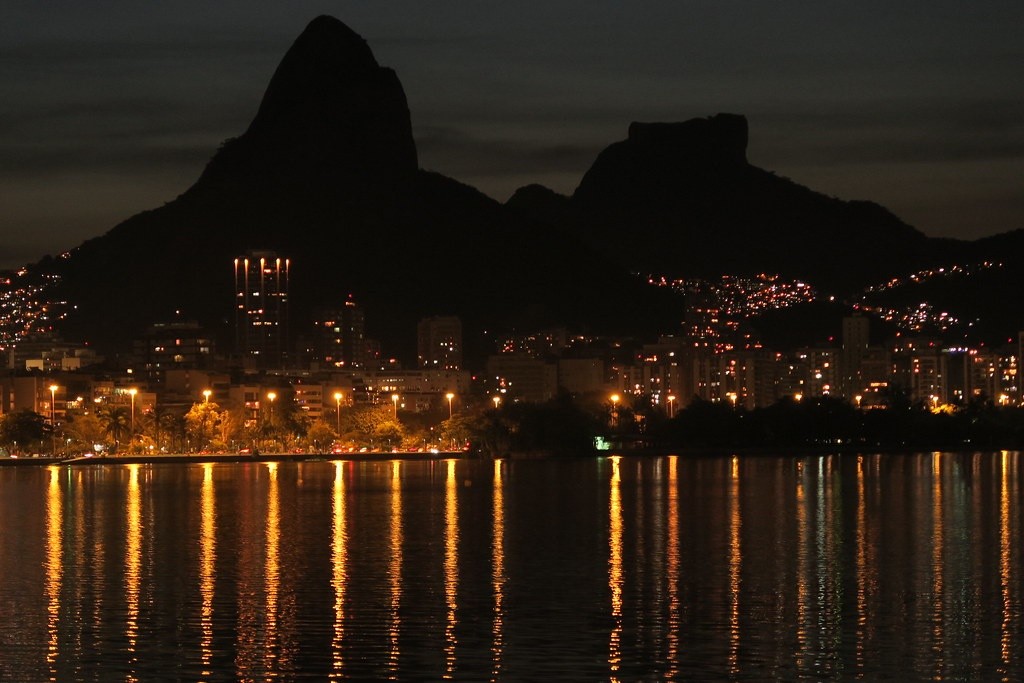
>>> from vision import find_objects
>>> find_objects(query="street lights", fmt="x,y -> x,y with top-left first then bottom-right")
334,391 -> 343,434
668,396 -> 675,418
268,393 -> 277,421
12,437 -> 191,453
128,388 -> 138,435
855,395 -> 863,408
611,395 -> 618,426
445,393 -> 454,418
202,388 -> 213,404
391,394 -> 399,418
492,397 -> 501,408
933,396 -> 939,408
48,385 -> 59,456
370,435 -> 472,452
730,395 -> 738,408
